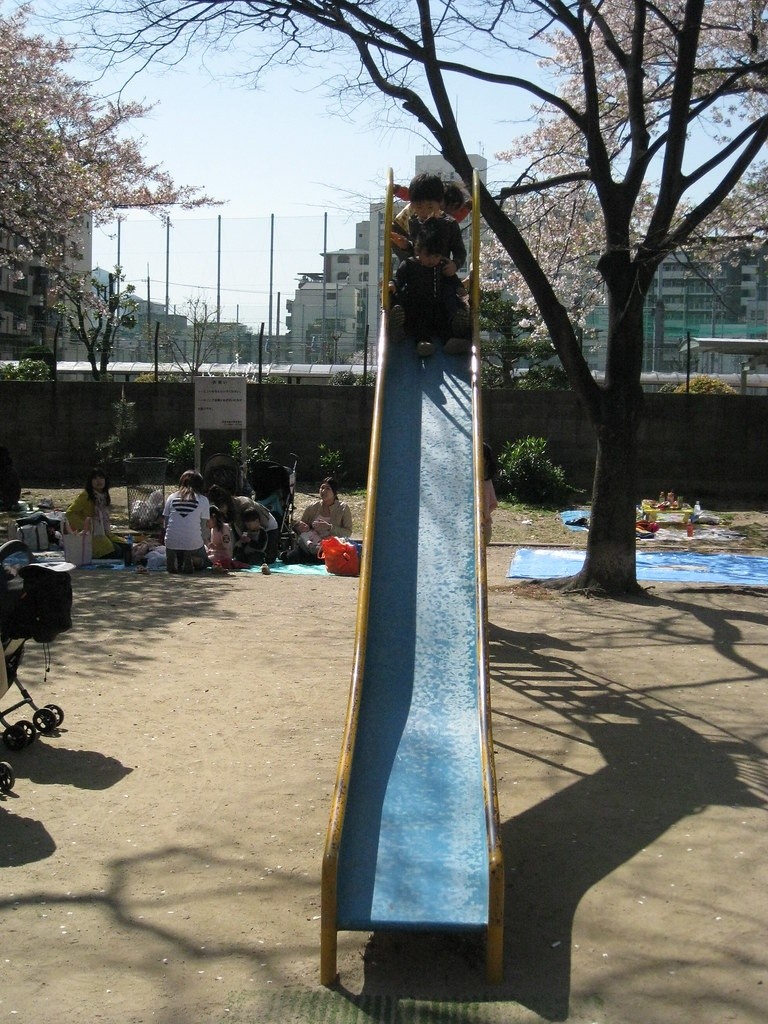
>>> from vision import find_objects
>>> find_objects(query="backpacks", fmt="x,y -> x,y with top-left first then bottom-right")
15,561 -> 77,682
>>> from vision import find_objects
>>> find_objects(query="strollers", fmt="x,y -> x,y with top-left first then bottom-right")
230,449 -> 301,567
0,539 -> 77,791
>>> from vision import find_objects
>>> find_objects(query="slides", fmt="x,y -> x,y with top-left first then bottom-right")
320,169 -> 505,990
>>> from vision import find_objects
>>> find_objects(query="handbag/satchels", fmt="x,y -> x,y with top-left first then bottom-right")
317,536 -> 360,576
59,516 -> 93,566
15,520 -> 49,552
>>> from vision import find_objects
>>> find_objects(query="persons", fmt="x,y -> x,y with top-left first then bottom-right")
292,521 -> 330,555
210,483 -> 279,564
278,475 -> 354,564
162,471 -> 211,571
235,510 -> 264,565
379,225 -> 471,355
206,506 -> 248,568
480,439 -> 499,546
391,173 -> 468,276
65,469 -> 128,559
390,181 -> 474,264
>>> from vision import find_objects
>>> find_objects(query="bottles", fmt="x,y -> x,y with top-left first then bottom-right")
636,490 -> 702,539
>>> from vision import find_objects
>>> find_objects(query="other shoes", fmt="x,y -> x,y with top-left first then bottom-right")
280,551 -> 290,559
219,558 -> 249,570
265,543 -> 278,564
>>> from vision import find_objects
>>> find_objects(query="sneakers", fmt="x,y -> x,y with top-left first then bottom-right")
190,550 -> 204,573
416,337 -> 434,357
441,337 -> 469,354
169,550 -> 185,572
449,309 -> 470,338
389,304 -> 406,344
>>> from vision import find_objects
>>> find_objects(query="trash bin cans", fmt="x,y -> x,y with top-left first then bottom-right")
124,456 -> 169,532
